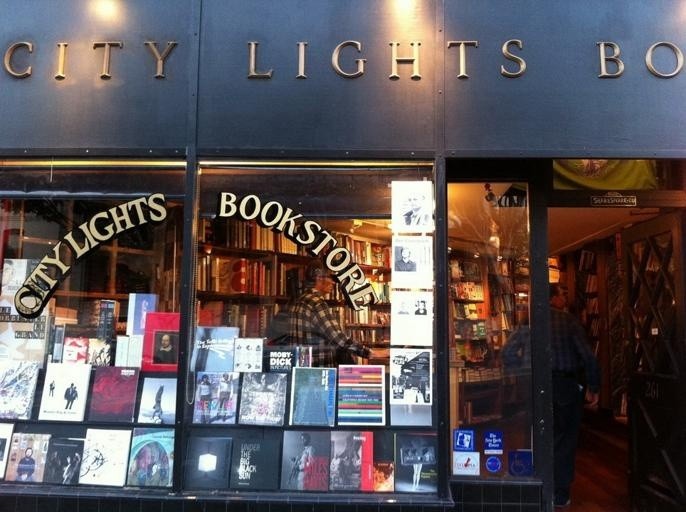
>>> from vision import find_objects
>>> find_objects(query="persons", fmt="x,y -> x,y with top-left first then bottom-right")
499,281 -> 603,509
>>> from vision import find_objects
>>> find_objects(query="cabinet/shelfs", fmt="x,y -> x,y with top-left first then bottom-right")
19,195 -> 629,432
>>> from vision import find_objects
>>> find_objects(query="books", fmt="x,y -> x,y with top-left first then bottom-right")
0,195 -> 675,496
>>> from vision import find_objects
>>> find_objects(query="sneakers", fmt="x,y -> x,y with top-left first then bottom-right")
554,487 -> 571,507
